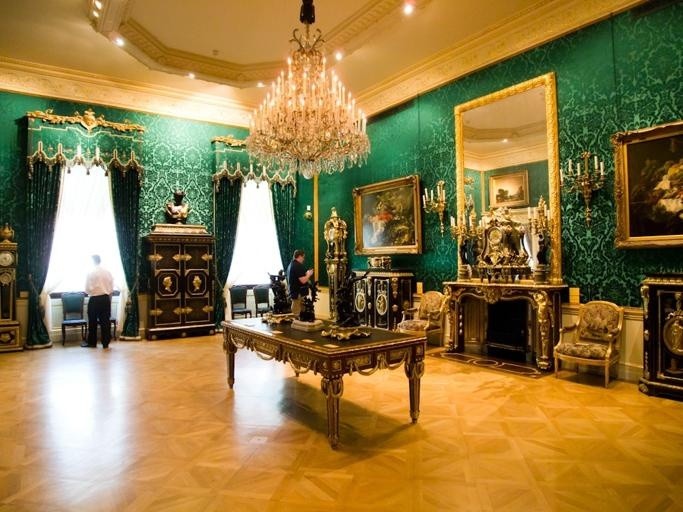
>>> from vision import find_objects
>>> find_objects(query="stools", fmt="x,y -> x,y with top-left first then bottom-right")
90,319 -> 118,341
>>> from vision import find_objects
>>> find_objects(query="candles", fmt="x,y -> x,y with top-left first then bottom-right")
528,206 -> 552,220
449,215 -> 474,228
423,184 -> 447,207
560,155 -> 605,181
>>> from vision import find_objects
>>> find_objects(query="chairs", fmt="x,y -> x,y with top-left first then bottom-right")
60,291 -> 87,346
252,286 -> 272,317
229,286 -> 252,320
394,290 -> 448,349
551,301 -> 623,386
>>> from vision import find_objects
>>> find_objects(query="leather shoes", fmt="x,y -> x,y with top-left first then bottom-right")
82,343 -> 96,348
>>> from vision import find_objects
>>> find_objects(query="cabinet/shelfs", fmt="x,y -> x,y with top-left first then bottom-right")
144,234 -> 214,336
347,271 -> 412,325
637,281 -> 682,394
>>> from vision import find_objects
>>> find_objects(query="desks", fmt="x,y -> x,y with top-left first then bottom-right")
220,311 -> 427,449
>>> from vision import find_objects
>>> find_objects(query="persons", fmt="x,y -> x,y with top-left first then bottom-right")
82,253 -> 114,348
163,277 -> 173,293
193,275 -> 207,293
287,250 -> 314,315
166,191 -> 190,221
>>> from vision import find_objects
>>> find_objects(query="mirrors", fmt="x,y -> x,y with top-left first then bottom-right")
453,72 -> 562,285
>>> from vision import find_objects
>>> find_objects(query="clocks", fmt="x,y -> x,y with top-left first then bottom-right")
322,206 -> 346,259
0,223 -> 17,321
477,217 -> 511,282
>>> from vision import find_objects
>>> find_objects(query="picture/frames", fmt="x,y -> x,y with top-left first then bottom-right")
350,175 -> 422,255
487,170 -> 530,208
610,120 -> 682,249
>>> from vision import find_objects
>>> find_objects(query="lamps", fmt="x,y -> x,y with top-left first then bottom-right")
246,2 -> 370,175
